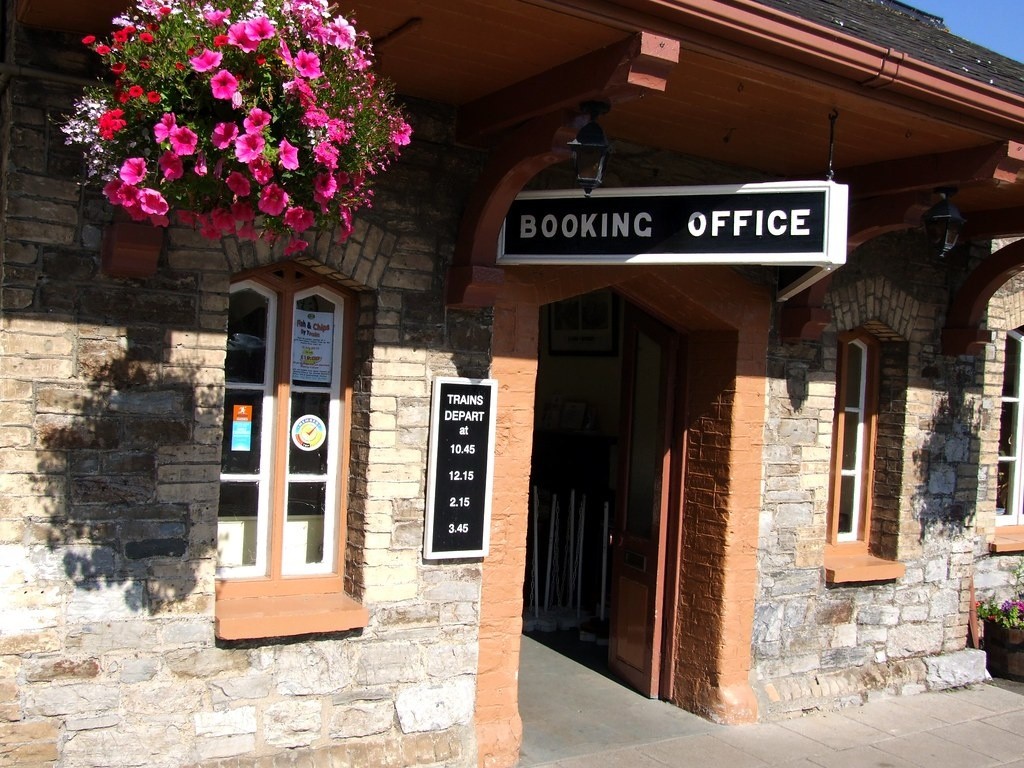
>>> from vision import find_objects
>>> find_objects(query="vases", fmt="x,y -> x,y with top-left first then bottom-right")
983,622 -> 1024,683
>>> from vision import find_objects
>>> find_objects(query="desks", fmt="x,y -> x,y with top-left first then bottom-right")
526,427 -> 618,612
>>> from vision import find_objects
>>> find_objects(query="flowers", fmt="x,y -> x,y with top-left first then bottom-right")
975,557 -> 1024,632
47,0 -> 415,258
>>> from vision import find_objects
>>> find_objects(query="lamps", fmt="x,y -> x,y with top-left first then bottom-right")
920,185 -> 968,263
566,98 -> 614,198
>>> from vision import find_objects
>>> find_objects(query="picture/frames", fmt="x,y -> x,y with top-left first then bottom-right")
548,288 -> 619,357
